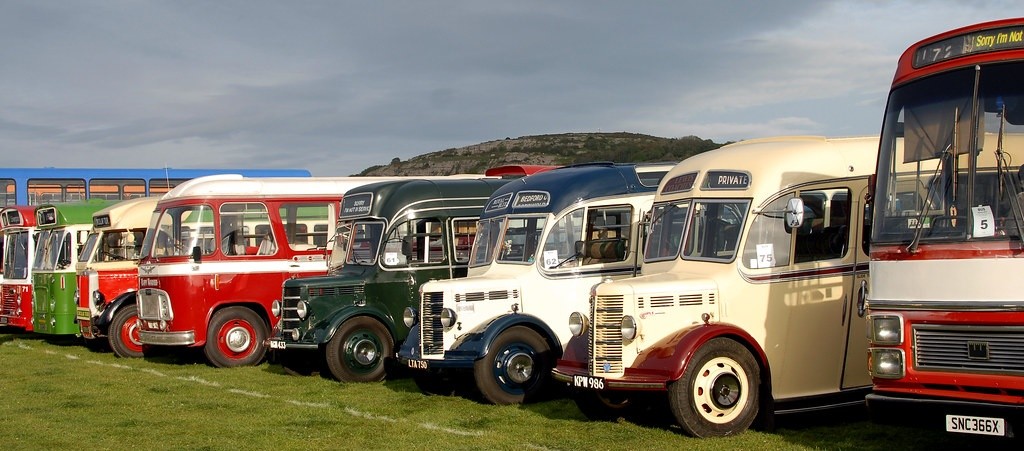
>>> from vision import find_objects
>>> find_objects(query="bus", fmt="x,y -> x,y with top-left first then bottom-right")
550,128 -> 1023,438
0,204 -> 43,331
74,194 -> 191,358
7,183 -> 176,207
136,164 -> 563,367
1,166 -> 312,217
395,161 -> 742,405
261,180 -> 629,386
865,14 -> 1024,435
33,197 -> 328,335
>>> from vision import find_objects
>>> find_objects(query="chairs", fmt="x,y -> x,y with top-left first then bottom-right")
583,237 -> 626,265
822,224 -> 870,258
